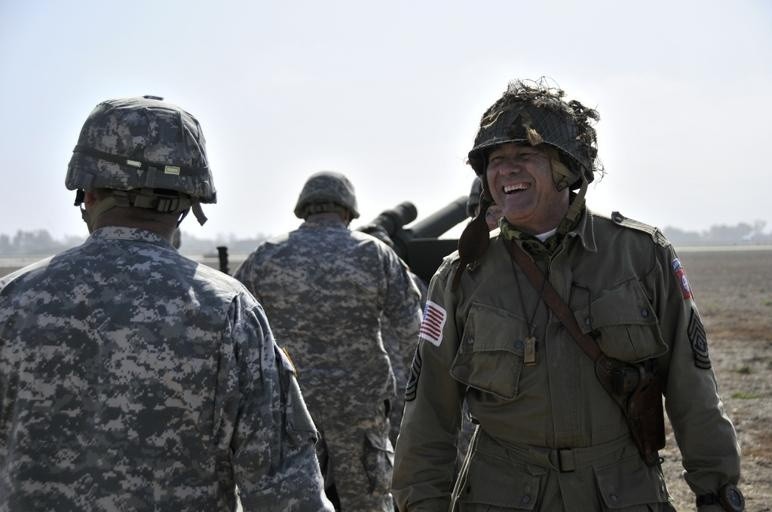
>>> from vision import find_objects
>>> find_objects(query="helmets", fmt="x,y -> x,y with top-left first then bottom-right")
466,90 -> 594,217
65,95 -> 217,205
294,172 -> 360,219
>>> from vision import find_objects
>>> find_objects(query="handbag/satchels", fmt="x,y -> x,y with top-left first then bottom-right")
594,360 -> 667,468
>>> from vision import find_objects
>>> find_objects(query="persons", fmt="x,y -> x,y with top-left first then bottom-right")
391,83 -> 745,512
0,94 -> 335,511
232,171 -> 423,510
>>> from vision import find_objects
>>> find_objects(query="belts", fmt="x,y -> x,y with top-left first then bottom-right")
509,433 -> 641,475
